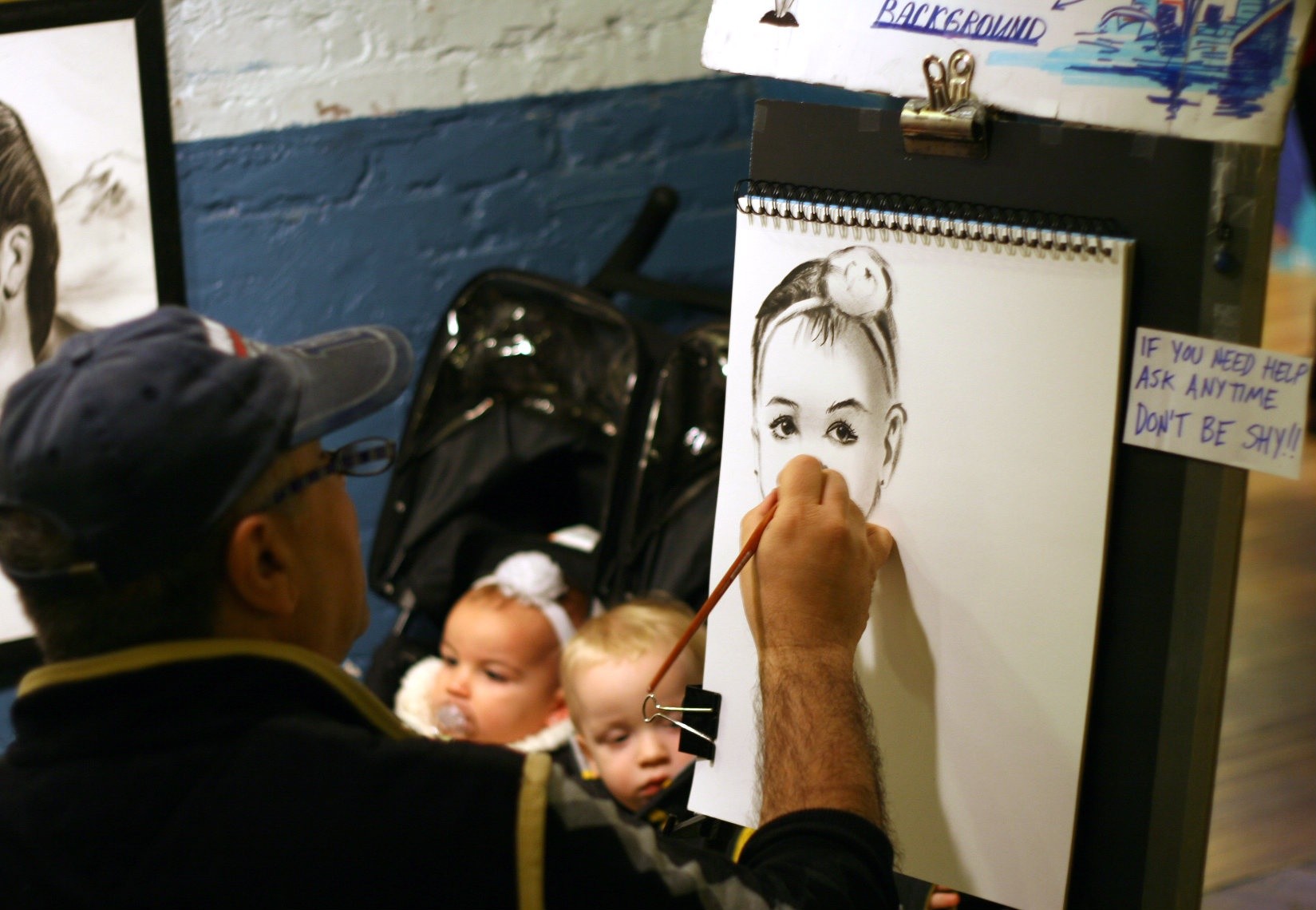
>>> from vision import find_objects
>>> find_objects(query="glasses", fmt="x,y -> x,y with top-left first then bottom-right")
265,436 -> 397,508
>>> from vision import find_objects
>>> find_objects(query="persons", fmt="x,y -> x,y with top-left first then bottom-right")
2,307 -> 896,910
383,549 -> 610,798
559,588 -> 769,910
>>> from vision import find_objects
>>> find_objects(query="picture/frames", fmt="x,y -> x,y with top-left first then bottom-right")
0,2 -> 188,690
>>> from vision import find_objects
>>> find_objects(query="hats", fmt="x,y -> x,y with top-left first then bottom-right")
0,307 -> 415,575
